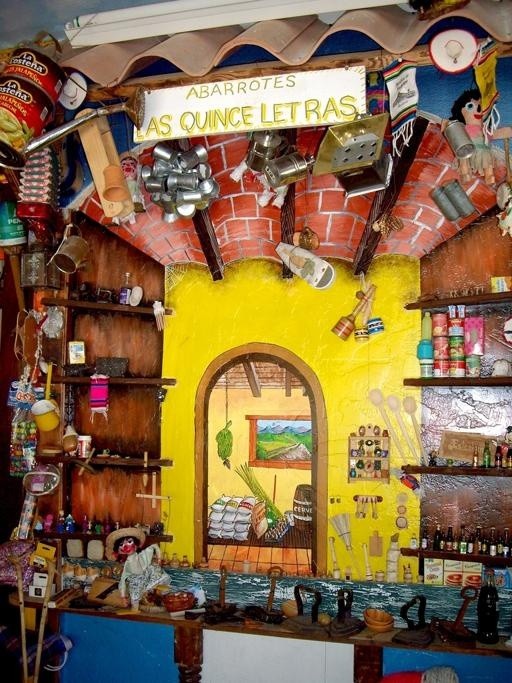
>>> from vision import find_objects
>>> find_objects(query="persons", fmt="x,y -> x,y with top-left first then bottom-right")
106,528 -> 172,612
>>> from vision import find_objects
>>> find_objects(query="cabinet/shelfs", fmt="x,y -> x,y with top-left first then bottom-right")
34,294 -> 177,557
400,290 -> 512,568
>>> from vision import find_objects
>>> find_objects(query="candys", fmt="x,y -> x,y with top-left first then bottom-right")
9,422 -> 37,477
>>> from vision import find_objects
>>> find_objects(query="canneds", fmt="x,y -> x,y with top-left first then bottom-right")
432,313 -> 481,378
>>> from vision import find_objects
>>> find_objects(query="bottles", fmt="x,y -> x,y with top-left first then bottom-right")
280,599 -> 299,619
420,521 -> 512,557
411,533 -> 417,549
56,510 -> 122,537
347,424 -> 390,480
473,437 -> 512,470
421,311 -> 433,341
417,340 -> 433,360
476,569 -> 501,645
120,272 -> 132,306
161,552 -> 209,570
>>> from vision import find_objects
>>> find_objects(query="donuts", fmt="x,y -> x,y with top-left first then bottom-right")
445,574 -> 482,589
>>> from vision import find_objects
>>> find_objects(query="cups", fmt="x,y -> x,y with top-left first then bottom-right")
430,187 -> 460,221
30,400 -> 61,432
444,120 -> 476,161
443,179 -> 476,219
77,435 -> 92,458
55,234 -> 90,274
140,143 -> 220,224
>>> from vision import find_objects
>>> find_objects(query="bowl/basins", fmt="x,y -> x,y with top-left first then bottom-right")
163,592 -> 196,612
364,608 -> 394,633
